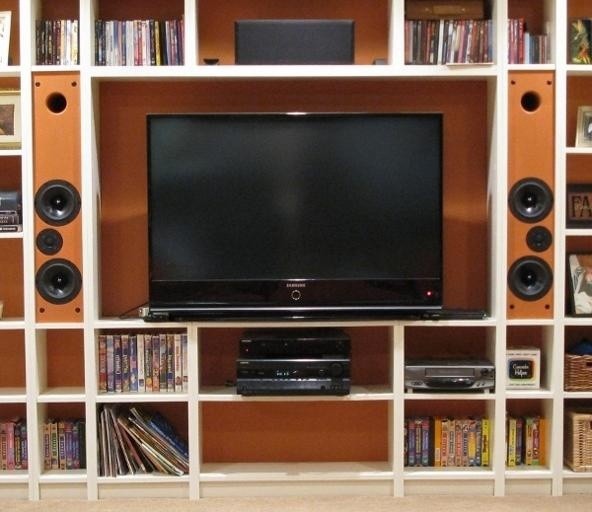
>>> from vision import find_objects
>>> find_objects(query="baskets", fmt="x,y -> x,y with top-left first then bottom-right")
564,406 -> 592,472
564,353 -> 592,392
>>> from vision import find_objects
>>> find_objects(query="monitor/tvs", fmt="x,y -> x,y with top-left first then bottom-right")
145,112 -> 444,315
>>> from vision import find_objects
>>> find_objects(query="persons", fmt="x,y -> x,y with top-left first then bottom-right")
573,253 -> 592,298
586,117 -> 592,140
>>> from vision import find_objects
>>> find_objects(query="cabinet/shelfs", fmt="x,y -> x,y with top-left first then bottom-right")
0,0 -> 592,502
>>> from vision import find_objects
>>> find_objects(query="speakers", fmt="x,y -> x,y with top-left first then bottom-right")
233,18 -> 354,65
507,71 -> 554,319
33,72 -> 82,324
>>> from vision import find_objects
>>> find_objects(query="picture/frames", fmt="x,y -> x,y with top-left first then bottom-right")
0,90 -> 22,150
566,184 -> 592,229
574,105 -> 592,147
566,250 -> 592,317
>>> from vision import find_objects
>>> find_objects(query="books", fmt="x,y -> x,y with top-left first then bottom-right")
1,414 -> 86,470
96,332 -> 191,477
1,185 -> 23,233
403,18 -> 553,65
35,18 -> 184,67
404,411 -> 546,467
